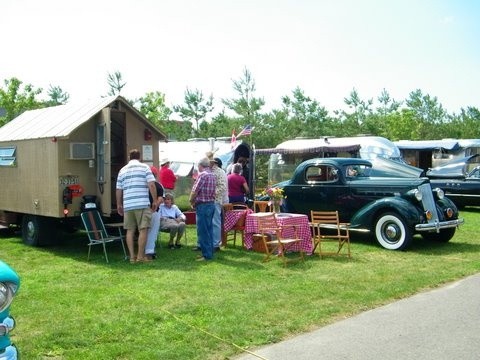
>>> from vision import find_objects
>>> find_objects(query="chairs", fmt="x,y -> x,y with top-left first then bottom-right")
222,203 -> 246,249
81,210 -> 128,263
254,201 -> 271,213
157,229 -> 188,249
308,210 -> 351,259
257,212 -> 305,265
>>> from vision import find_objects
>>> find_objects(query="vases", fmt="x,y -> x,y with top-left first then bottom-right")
273,201 -> 280,214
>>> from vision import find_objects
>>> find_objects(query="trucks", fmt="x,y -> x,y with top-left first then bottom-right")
161,135 -> 480,204
0,95 -> 166,246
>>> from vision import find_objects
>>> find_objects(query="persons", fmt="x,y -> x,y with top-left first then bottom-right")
159,160 -> 177,194
159,193 -> 186,249
143,172 -> 165,261
116,150 -> 158,263
189,151 -> 249,261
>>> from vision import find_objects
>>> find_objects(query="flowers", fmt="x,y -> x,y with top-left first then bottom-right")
261,187 -> 288,205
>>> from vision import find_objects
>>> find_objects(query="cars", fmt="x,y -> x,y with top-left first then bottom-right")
256,157 -> 464,249
427,153 -> 480,210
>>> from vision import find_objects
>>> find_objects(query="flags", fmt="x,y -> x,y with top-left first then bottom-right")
231,125 -> 251,149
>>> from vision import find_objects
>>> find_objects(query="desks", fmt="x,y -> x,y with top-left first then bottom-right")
246,214 -> 313,255
104,224 -> 128,262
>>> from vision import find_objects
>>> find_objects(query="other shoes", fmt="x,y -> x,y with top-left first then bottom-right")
128,254 -> 153,263
176,242 -> 183,248
213,247 -> 220,253
168,242 -> 174,250
195,256 -> 207,262
192,247 -> 202,251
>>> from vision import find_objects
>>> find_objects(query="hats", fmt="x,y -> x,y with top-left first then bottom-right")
207,152 -> 215,162
